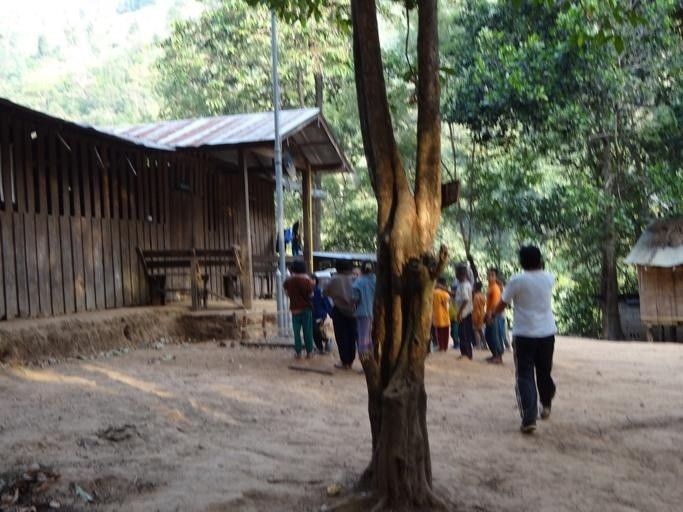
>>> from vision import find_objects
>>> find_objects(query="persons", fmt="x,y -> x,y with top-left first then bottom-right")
282,258 -> 315,360
308,257 -> 514,370
486,244 -> 556,434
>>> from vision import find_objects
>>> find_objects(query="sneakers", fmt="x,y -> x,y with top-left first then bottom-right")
521,420 -> 536,431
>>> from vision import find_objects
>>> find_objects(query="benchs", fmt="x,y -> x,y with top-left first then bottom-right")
135,247 -> 305,305
